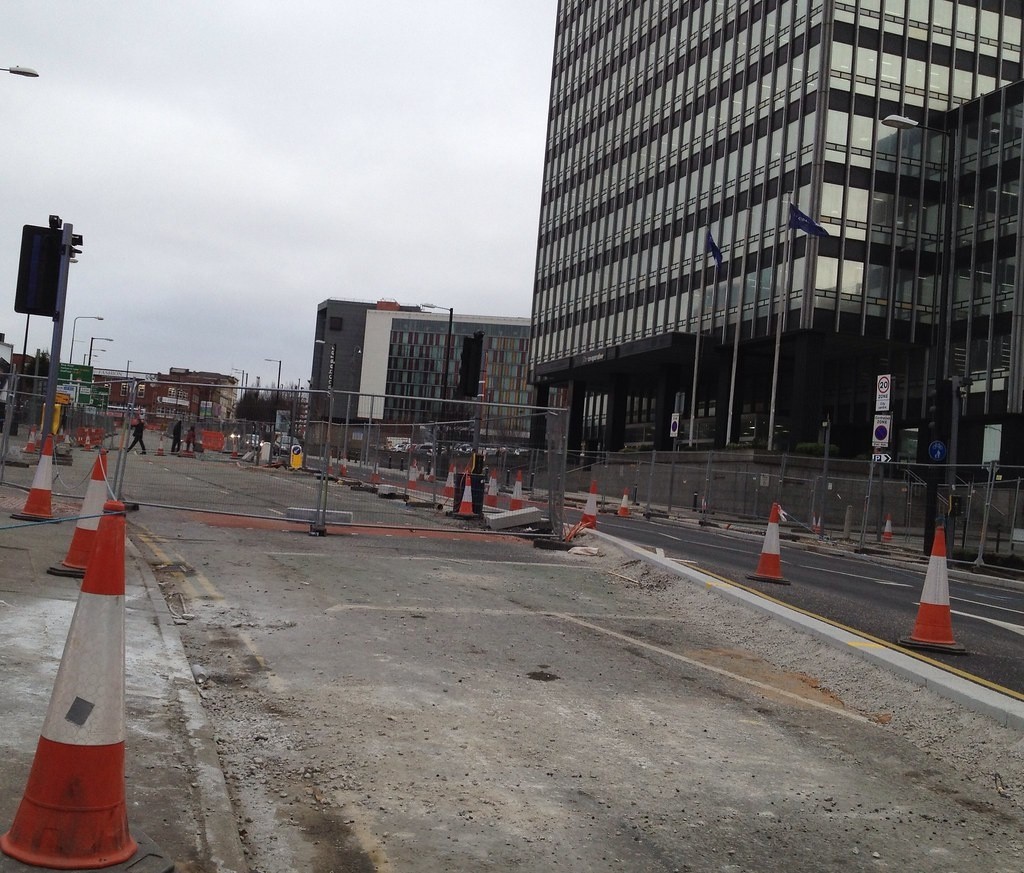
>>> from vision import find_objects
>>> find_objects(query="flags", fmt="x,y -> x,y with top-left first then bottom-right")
789,203 -> 829,236
707,232 -> 722,267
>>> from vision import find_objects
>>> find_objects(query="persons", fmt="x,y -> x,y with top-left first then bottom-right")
186,426 -> 195,451
171,421 -> 182,452
124,419 -> 146,454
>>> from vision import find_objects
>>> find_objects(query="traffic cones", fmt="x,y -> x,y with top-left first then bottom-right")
899,524 -> 968,654
1,448 -> 176,870
10,433 -> 62,524
881,513 -> 895,542
579,479 -> 598,531
154,436 -> 167,456
327,450 -> 348,478
366,460 -> 381,484
406,459 -> 435,490
19,423 -> 105,455
441,461 -> 524,517
229,441 -> 241,459
745,501 -> 792,585
812,512 -> 827,535
180,437 -> 196,458
615,487 -> 634,518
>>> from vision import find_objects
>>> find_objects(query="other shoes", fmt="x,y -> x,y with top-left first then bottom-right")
171,453 -> 176,455
140,452 -> 146,455
127,451 -> 129,453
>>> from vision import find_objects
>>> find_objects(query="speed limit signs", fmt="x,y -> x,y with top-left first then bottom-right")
875,374 -> 891,411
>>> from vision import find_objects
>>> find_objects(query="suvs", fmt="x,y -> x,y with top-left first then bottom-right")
240,434 -> 264,451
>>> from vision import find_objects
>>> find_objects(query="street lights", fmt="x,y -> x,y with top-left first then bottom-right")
70,316 -> 104,364
232,369 -> 260,421
83,337 -> 114,366
266,360 -> 281,422
126,360 -> 133,378
477,380 -> 493,450
315,339 -> 336,456
881,114 -> 958,556
420,303 -> 453,480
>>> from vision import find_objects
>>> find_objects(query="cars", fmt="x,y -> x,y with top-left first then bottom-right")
273,436 -> 303,456
